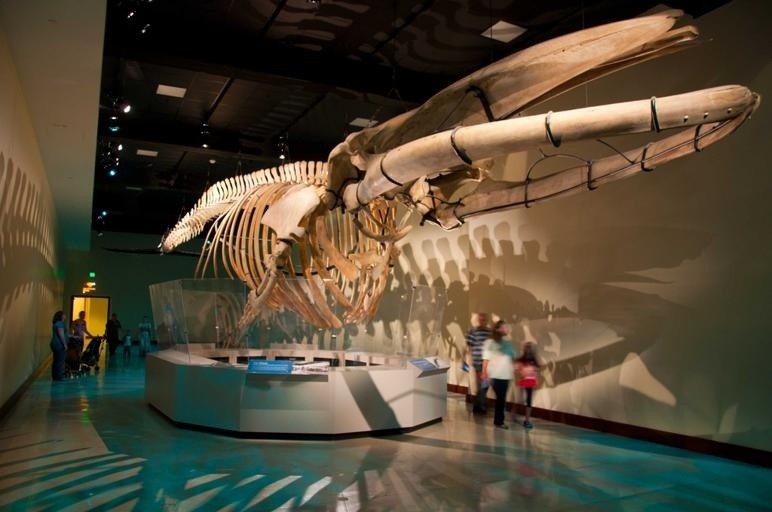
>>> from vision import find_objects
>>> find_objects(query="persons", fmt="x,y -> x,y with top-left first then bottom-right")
476,430 -> 537,511
103,312 -> 121,358
71,310 -> 93,349
479,319 -> 517,430
121,329 -> 133,360
463,312 -> 494,416
49,310 -> 70,382
513,340 -> 542,429
137,314 -> 152,357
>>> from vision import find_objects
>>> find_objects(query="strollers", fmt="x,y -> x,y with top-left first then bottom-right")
79,336 -> 105,371
